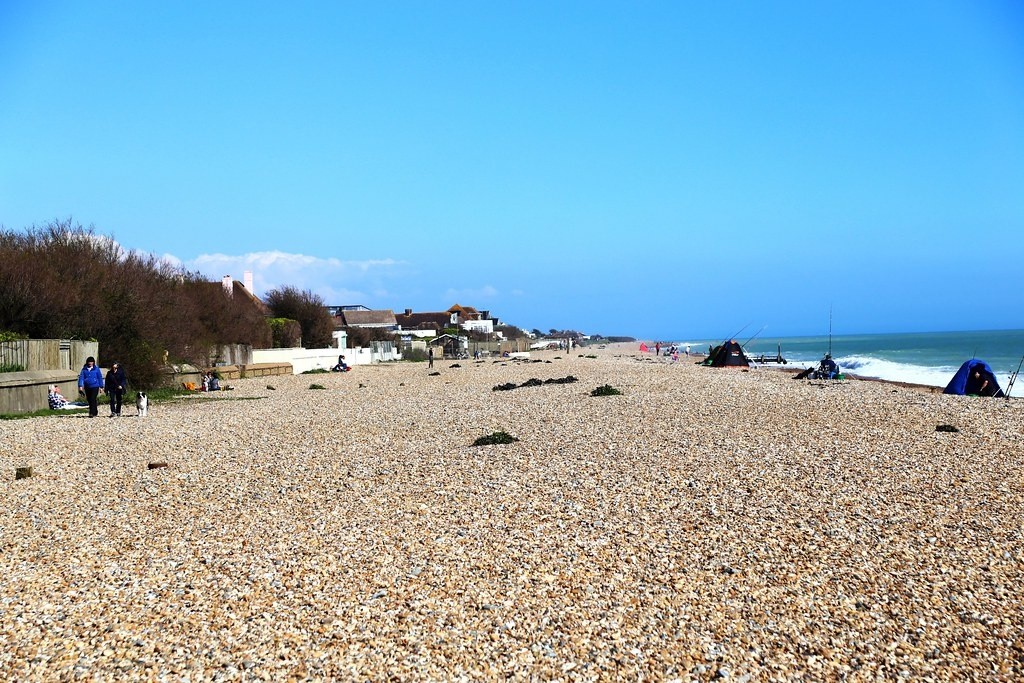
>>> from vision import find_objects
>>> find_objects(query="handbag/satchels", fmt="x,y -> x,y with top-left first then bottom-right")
121,387 -> 126,395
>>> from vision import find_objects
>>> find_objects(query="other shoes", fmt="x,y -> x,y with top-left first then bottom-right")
111,412 -> 115,416
117,413 -> 121,416
89,414 -> 93,418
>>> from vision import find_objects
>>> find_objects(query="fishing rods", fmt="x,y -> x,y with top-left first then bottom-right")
719,320 -> 764,350
991,354 -> 1024,401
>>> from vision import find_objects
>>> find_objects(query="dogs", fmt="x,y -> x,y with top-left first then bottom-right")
136,392 -> 148,416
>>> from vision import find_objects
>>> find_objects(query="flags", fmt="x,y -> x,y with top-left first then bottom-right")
450,313 -> 458,324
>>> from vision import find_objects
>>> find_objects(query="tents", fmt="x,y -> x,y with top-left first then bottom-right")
429,334 -> 464,355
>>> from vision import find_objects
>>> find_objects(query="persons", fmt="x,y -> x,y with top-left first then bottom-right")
656,341 -> 714,356
202,371 -> 234,391
548,340 -> 576,350
974,369 -> 996,396
429,347 -> 509,369
821,355 -> 840,379
103,362 -> 127,417
48,385 -> 89,411
77,357 -> 103,418
